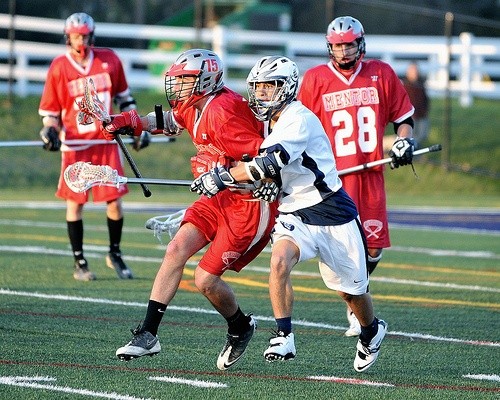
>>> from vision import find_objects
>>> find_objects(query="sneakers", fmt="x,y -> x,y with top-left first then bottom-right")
345,314 -> 362,337
353,318 -> 388,373
262,326 -> 296,362
217,312 -> 256,370
105,251 -> 131,279
116,324 -> 161,362
73,261 -> 95,281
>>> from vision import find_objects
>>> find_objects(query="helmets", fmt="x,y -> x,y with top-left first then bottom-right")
64,13 -> 95,44
164,49 -> 224,115
246,55 -> 299,120
326,16 -> 365,68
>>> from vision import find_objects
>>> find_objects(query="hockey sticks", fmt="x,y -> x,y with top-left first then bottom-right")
77,76 -> 150,198
145,145 -> 444,233
63,161 -> 257,195
1,137 -> 178,146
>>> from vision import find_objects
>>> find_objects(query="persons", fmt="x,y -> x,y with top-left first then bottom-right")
99,49 -> 275,371
39,12 -> 149,282
190,55 -> 387,373
403,65 -> 430,147
297,16 -> 416,335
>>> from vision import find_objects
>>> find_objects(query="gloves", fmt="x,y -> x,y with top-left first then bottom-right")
250,179 -> 279,203
40,124 -> 62,152
191,153 -> 229,178
131,131 -> 150,150
190,164 -> 235,199
387,137 -> 415,169
100,109 -> 142,140
